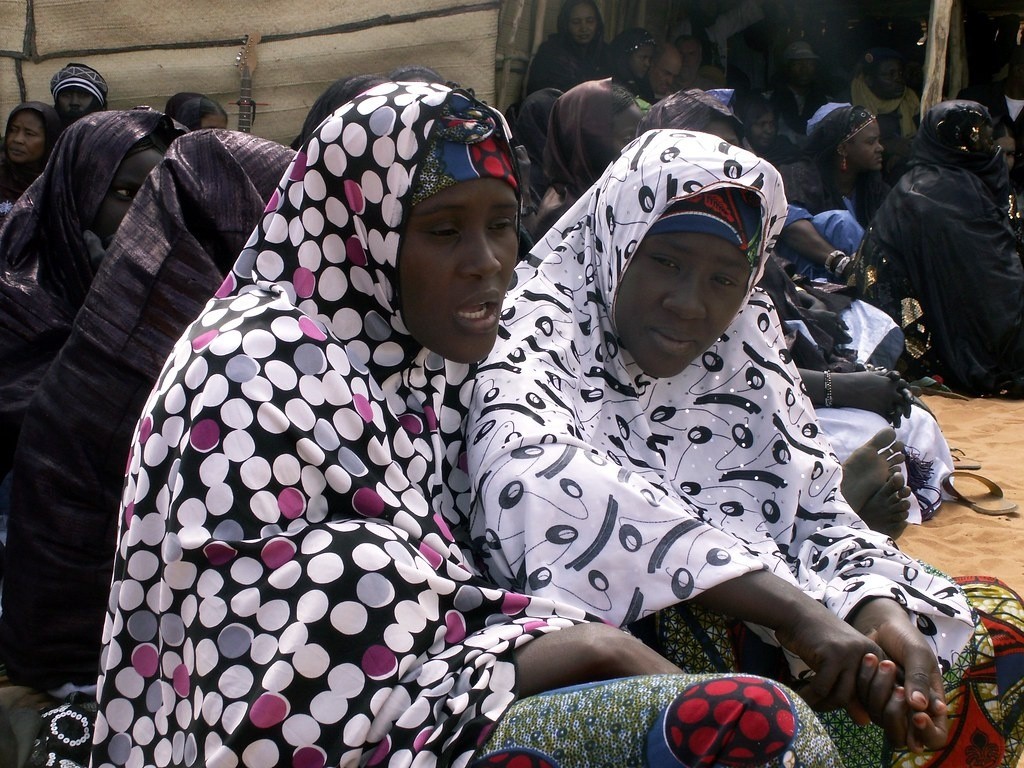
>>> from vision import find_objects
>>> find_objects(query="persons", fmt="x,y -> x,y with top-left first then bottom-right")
1,126 -> 303,703
47,62 -> 110,134
0,105 -> 198,603
0,685 -> 59,768
80,76 -> 851,768
0,100 -> 64,229
164,91 -> 229,135
288,0 -> 1024,544
464,127 -> 1024,768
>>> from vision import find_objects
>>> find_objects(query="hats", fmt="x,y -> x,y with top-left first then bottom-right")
778,40 -> 819,61
